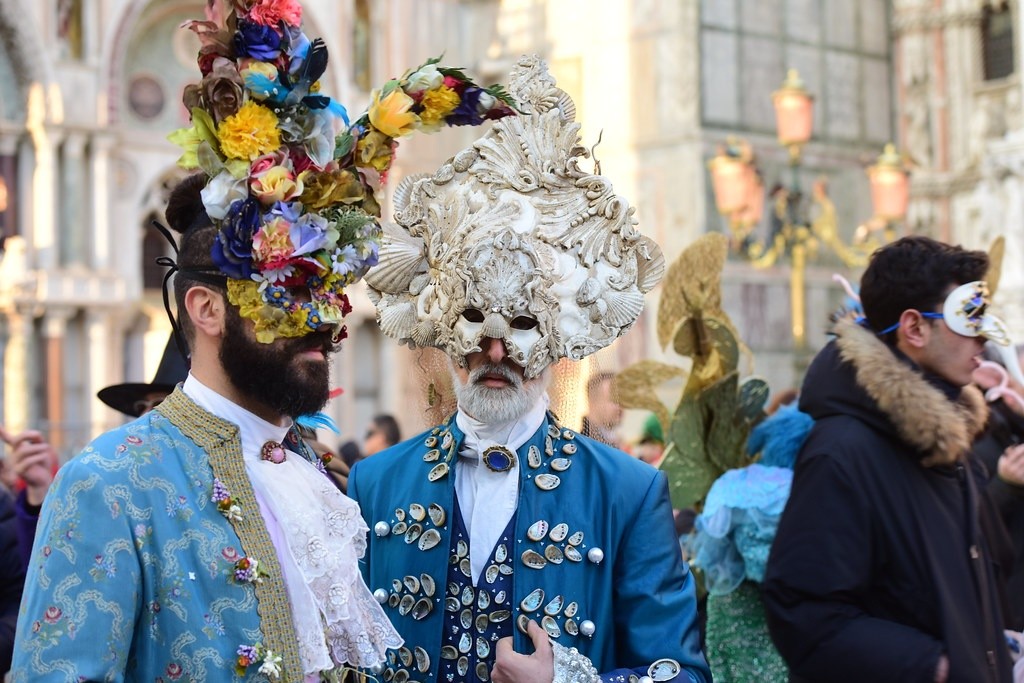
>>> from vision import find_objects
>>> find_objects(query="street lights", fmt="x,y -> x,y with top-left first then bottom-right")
702,67 -> 920,372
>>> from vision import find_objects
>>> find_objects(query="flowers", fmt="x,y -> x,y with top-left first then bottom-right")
166,1 -> 534,344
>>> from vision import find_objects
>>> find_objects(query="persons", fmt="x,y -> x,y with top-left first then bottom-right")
98,319 -> 401,490
579,372 -> 666,466
0,429 -> 50,683
347,251 -> 709,683
693,237 -> 1024,683
12,205 -> 406,683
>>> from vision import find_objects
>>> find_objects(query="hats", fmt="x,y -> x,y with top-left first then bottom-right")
97,320 -> 192,418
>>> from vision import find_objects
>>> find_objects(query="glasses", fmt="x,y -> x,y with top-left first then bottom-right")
133,398 -> 166,414
